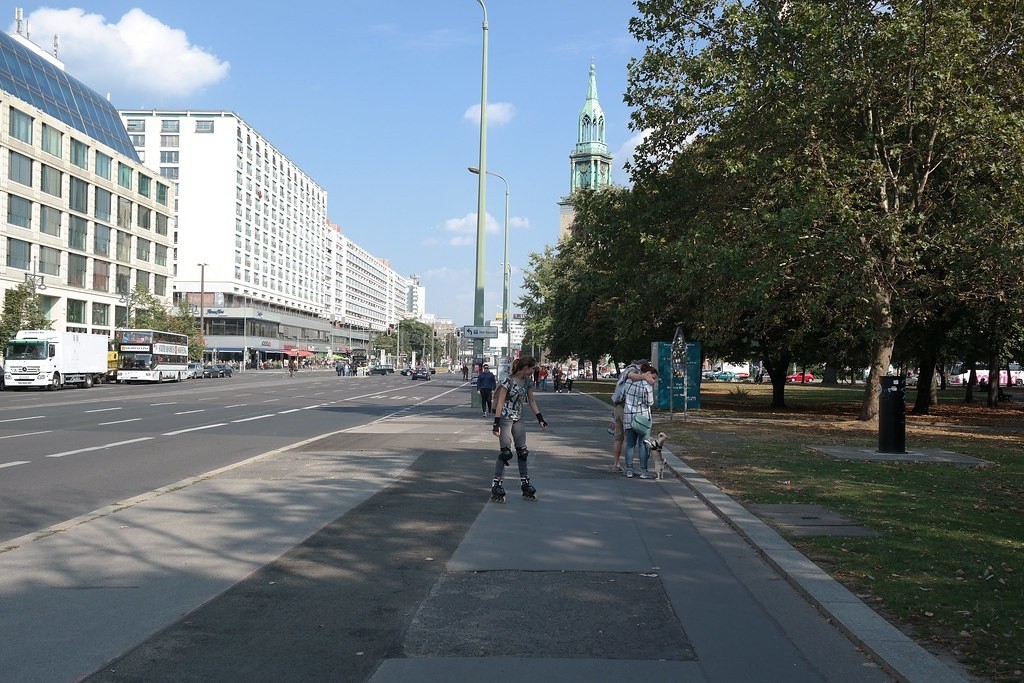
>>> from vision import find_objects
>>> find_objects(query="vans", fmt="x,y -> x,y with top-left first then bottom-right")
365,364 -> 395,376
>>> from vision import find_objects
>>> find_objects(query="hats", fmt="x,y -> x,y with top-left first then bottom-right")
633,358 -> 650,368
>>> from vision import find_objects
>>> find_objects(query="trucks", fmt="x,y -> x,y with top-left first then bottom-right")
2,329 -> 108,392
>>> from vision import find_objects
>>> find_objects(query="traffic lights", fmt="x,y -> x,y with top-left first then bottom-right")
386,327 -> 392,337
457,330 -> 461,339
433,329 -> 437,337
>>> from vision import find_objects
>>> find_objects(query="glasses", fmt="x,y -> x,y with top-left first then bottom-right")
485,367 -> 488,369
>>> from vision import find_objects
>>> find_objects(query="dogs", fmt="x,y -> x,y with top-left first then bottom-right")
644,432 -> 668,480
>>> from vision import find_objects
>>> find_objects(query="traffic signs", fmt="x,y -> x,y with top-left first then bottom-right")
464,325 -> 498,339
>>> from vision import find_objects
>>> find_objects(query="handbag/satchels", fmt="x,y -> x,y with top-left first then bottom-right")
608,413 -> 615,435
630,414 -> 652,435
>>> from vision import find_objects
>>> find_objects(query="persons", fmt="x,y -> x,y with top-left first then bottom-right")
534,364 -> 575,393
476,364 -> 497,416
462,364 -> 468,381
121,335 -> 371,378
491,356 -> 547,503
623,365 -> 658,479
610,360 -> 649,475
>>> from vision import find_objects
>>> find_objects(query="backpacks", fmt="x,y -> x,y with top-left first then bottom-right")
492,376 -> 513,414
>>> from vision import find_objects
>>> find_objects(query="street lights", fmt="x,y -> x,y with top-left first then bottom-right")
467,167 -> 512,386
197,263 -> 208,364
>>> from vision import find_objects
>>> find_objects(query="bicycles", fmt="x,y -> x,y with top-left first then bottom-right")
281,366 -> 301,379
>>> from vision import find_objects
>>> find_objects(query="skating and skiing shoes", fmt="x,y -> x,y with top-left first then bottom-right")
490,478 -> 506,504
520,476 -> 537,501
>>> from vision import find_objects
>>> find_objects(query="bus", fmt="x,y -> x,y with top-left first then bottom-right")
947,361 -> 1023,387
352,348 -> 368,371
117,328 -> 189,384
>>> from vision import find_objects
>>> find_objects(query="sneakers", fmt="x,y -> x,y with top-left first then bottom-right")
483,412 -> 487,417
613,464 -> 624,472
639,469 -> 654,479
627,467 -> 636,477
623,467 -> 627,475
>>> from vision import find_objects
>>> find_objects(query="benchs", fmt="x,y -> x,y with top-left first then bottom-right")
999,387 -> 1013,403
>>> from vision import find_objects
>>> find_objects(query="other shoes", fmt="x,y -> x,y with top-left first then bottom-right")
534,386 -> 574,393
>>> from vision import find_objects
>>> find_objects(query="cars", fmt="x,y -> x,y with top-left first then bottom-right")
203,364 -> 220,379
905,372 -> 941,387
786,372 -> 814,385
216,363 -> 233,378
412,366 -> 432,381
188,362 -> 205,380
700,369 -> 772,383
400,365 -> 436,377
